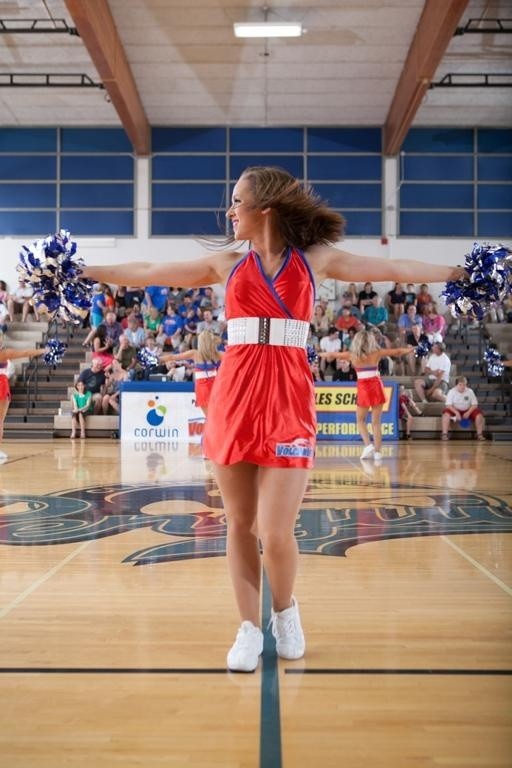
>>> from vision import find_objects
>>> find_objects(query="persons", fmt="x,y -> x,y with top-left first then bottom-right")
34,164 -> 509,672
490,301 -> 507,324
0,280 -> 227,439
316,331 -> 419,457
495,360 -> 509,366
306,280 -> 485,441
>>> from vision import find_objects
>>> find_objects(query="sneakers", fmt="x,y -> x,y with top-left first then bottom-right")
226,619 -> 265,673
227,673 -> 263,710
373,451 -> 384,460
266,593 -> 307,661
476,434 -> 486,441
360,460 -> 376,477
440,432 -> 449,441
360,443 -> 375,460
0,458 -> 8,463
374,461 -> 382,467
0,451 -> 8,458
417,412 -> 425,417
268,661 -> 306,703
405,433 -> 413,440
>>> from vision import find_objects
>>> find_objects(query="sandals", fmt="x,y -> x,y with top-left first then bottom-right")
80,341 -> 91,349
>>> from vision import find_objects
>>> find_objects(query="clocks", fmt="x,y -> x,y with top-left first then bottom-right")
316,274 -> 337,300
